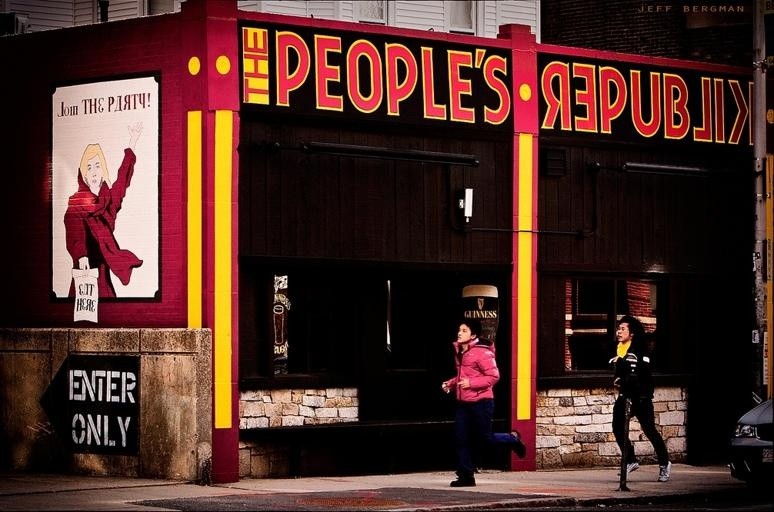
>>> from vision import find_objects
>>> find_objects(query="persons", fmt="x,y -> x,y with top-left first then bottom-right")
440,321 -> 526,487
607,315 -> 672,481
62,119 -> 144,303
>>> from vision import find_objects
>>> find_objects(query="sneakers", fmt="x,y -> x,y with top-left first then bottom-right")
510,430 -> 526,458
451,478 -> 476,487
657,461 -> 671,482
617,462 -> 640,476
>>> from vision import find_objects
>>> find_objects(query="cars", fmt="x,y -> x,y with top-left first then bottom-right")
726,393 -> 774,483
